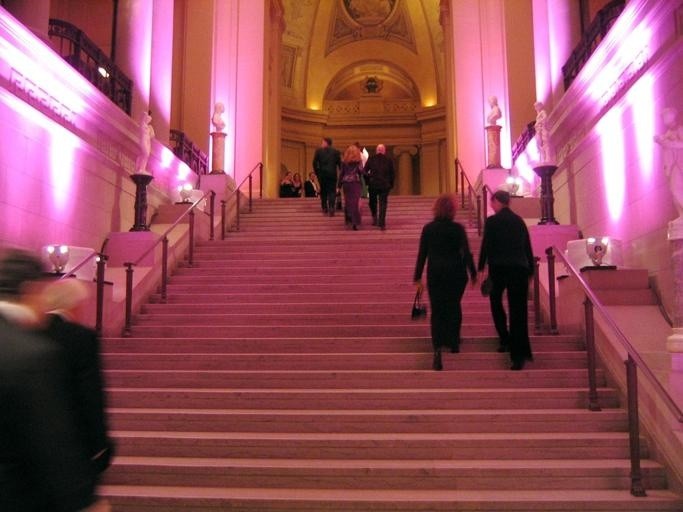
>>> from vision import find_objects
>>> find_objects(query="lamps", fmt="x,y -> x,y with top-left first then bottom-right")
178,182 -> 193,202
506,176 -> 523,195
586,236 -> 610,266
47,242 -> 69,273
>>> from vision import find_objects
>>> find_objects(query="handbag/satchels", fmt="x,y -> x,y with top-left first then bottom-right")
412,293 -> 427,320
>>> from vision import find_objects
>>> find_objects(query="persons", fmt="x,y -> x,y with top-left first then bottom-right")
312,137 -> 338,216
210,101 -> 225,131
411,197 -> 478,371
533,100 -> 549,163
487,96 -> 501,124
361,142 -> 395,232
40,273 -> 114,484
337,143 -> 364,233
278,171 -> 320,199
654,109 -> 683,218
475,190 -> 536,371
1,251 -> 92,509
133,112 -> 155,175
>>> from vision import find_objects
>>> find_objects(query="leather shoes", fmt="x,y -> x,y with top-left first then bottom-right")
433,338 -> 523,370
